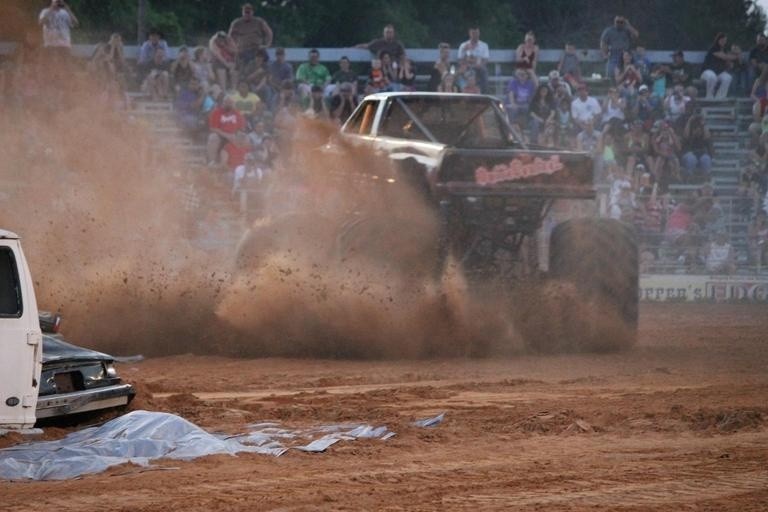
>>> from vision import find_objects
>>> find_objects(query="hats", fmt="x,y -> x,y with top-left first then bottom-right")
177,43 -> 413,121
512,44 -> 683,121
435,43 -> 489,85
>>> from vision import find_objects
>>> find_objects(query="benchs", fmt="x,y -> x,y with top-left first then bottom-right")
130,93 -> 768,275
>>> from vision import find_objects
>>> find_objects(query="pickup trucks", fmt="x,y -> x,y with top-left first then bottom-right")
234,91 -> 639,352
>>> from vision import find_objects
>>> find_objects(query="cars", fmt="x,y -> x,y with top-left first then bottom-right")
1,228 -> 137,436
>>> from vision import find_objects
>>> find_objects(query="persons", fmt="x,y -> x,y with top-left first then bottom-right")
24,3 -> 767,283
